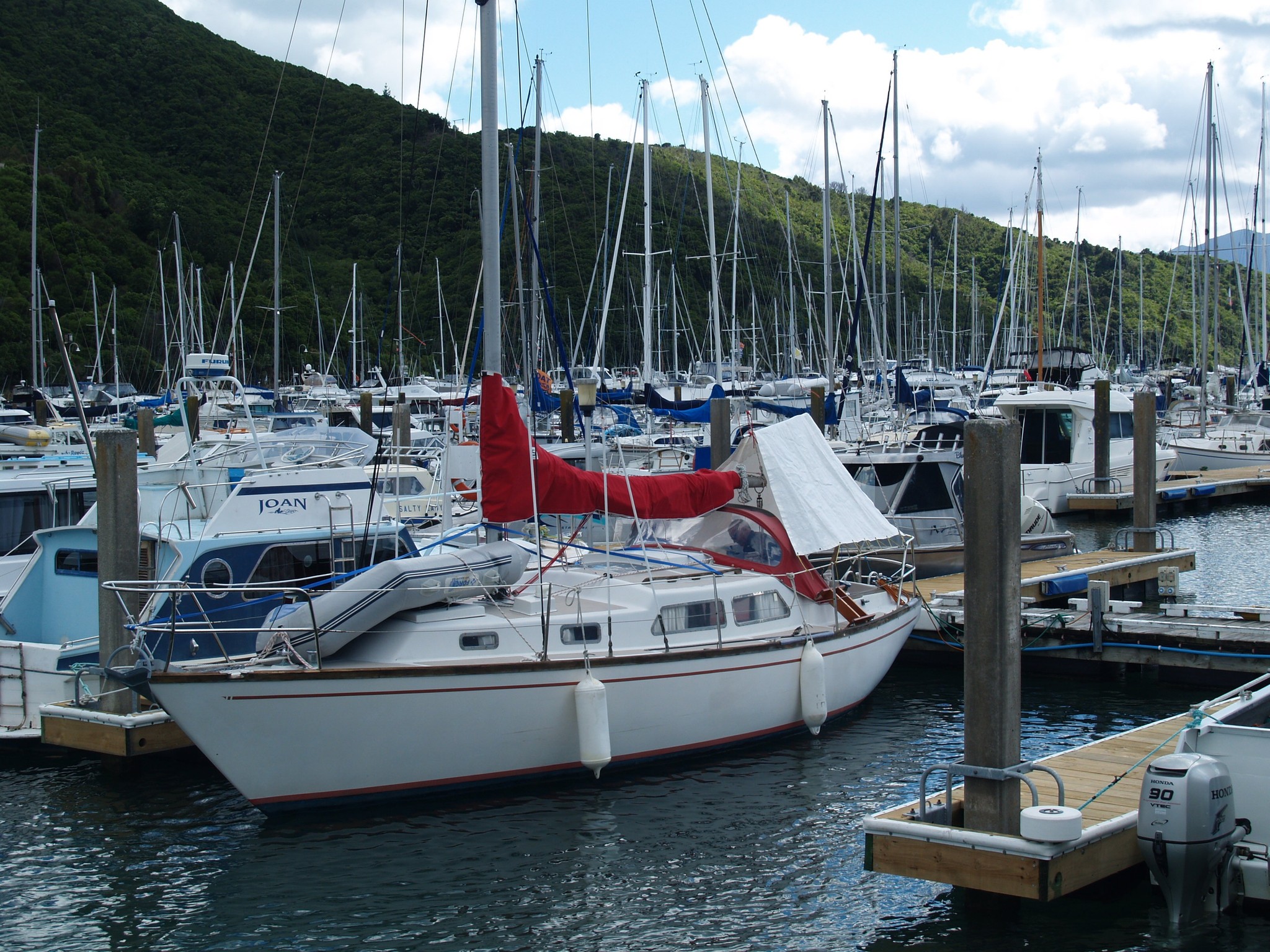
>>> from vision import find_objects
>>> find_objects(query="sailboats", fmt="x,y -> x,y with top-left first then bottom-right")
0,1 -> 1270,807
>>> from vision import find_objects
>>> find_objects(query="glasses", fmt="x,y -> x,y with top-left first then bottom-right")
732,528 -> 739,540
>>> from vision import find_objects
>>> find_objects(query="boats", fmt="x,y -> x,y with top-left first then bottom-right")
1135,673 -> 1270,942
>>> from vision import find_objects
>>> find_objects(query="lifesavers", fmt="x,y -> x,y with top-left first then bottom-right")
450,409 -> 466,432
451,441 -> 479,500
